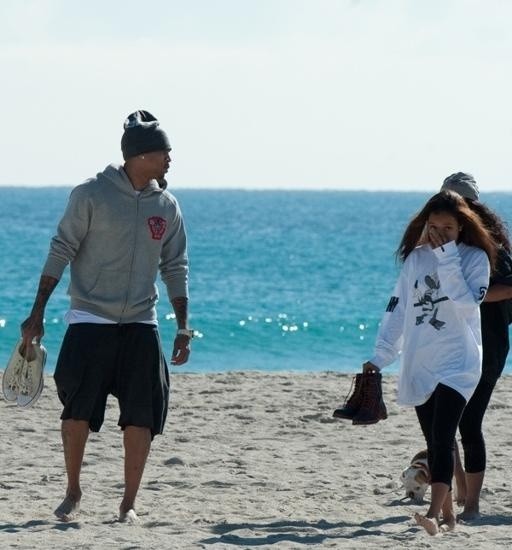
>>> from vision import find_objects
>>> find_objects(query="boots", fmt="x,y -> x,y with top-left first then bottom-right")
332,372 -> 388,426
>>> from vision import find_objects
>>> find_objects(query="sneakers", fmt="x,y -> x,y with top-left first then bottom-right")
0,338 -> 48,409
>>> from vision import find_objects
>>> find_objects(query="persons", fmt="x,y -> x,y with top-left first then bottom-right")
363,188 -> 497,536
440,172 -> 512,521
18,111 -> 189,525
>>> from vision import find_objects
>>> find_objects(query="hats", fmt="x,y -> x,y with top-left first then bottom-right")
121,109 -> 172,160
440,172 -> 481,199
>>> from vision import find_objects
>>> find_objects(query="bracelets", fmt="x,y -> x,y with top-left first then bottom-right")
177,329 -> 194,340
